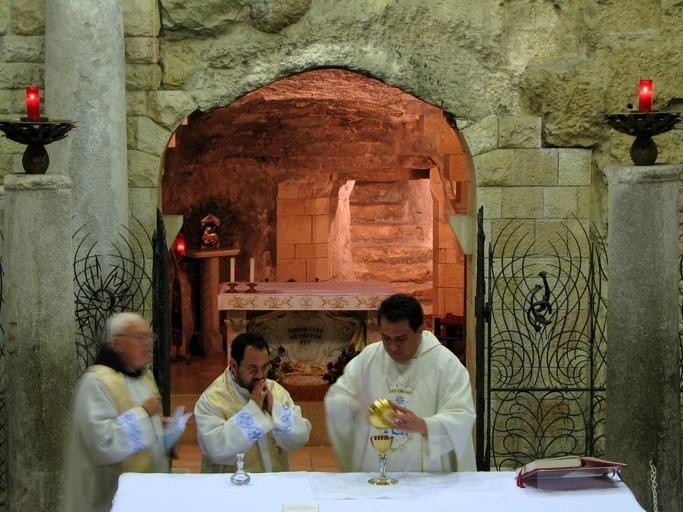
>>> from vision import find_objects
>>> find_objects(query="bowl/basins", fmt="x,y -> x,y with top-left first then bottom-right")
367,399 -> 398,430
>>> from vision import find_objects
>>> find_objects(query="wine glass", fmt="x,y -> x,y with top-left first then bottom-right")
368,435 -> 398,485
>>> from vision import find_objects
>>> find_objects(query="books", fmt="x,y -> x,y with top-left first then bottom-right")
514,453 -> 626,483
164,404 -> 193,452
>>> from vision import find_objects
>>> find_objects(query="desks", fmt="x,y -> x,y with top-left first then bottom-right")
110,470 -> 644,512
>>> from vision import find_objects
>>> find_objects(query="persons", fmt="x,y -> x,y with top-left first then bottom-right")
324,292 -> 477,473
61,311 -> 179,512
193,333 -> 312,471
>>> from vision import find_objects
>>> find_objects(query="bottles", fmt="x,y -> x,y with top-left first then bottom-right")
231,453 -> 250,486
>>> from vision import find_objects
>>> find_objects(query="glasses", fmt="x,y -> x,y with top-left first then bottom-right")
238,362 -> 273,375
114,332 -> 157,345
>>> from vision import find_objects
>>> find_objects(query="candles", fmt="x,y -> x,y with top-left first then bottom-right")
229,257 -> 235,283
639,79 -> 652,112
249,258 -> 256,283
25,85 -> 40,118
